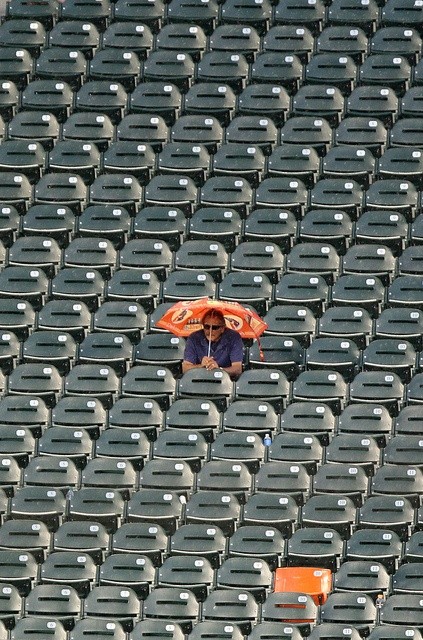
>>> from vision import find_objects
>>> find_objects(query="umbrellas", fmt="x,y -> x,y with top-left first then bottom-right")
157,296 -> 270,371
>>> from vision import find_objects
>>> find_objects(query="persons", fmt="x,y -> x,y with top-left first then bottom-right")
182,316 -> 246,380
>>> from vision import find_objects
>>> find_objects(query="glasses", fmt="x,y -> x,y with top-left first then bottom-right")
203,323 -> 222,330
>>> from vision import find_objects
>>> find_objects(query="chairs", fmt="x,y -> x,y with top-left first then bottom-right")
0,298 -> 423,397
1,3 -> 422,309
0,399 -> 421,639
